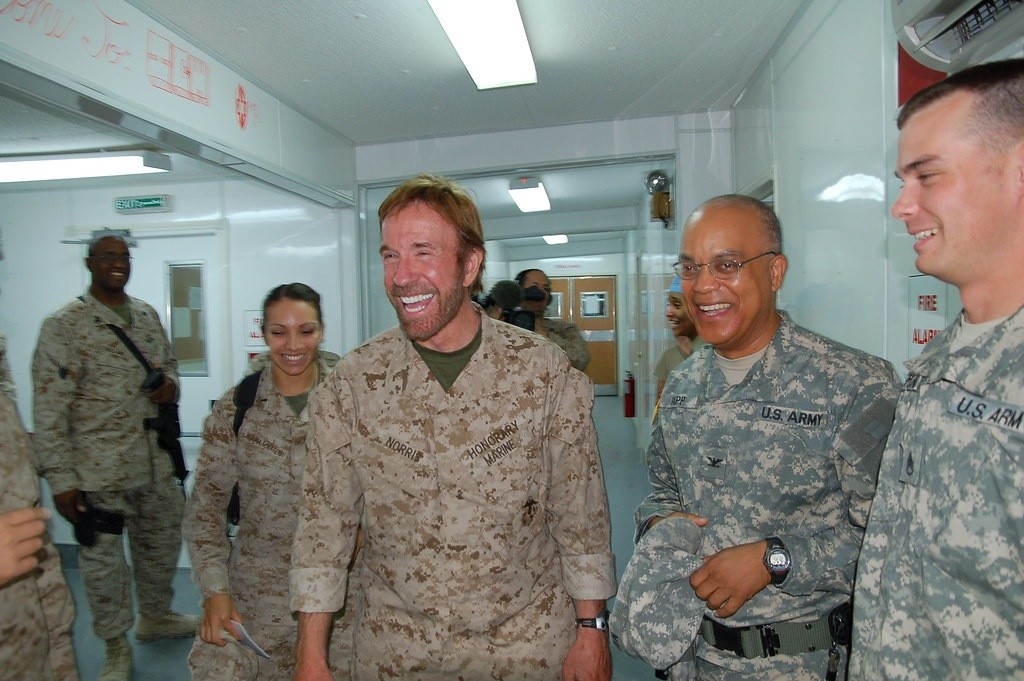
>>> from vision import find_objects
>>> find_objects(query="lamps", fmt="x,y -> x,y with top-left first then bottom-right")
508,177 -> 551,213
429,0 -> 539,90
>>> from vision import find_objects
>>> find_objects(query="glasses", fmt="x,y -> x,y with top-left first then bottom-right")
95,255 -> 132,266
672,251 -> 776,280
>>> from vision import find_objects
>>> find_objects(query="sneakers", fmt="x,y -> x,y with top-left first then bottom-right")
96,633 -> 132,681
134,612 -> 203,640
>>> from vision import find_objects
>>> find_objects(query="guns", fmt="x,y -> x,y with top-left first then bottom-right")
139,368 -> 189,486
210,400 -> 240,526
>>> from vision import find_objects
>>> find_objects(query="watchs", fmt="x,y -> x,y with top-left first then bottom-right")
573,609 -> 611,634
760,537 -> 791,586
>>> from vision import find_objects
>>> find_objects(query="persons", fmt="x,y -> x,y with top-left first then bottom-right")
31,235 -> 200,681
185,282 -> 367,681
848,56 -> 1024,681
653,276 -> 701,400
0,234 -> 83,681
627,195 -> 901,681
477,268 -> 592,372
288,171 -> 621,681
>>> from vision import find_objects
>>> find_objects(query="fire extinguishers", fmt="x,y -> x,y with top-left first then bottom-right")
623,369 -> 635,418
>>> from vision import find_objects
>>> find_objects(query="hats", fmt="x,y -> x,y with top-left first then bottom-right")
185,635 -> 279,681
609,516 -> 707,671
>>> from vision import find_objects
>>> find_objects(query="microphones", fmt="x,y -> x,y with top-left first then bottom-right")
491,279 -> 524,308
